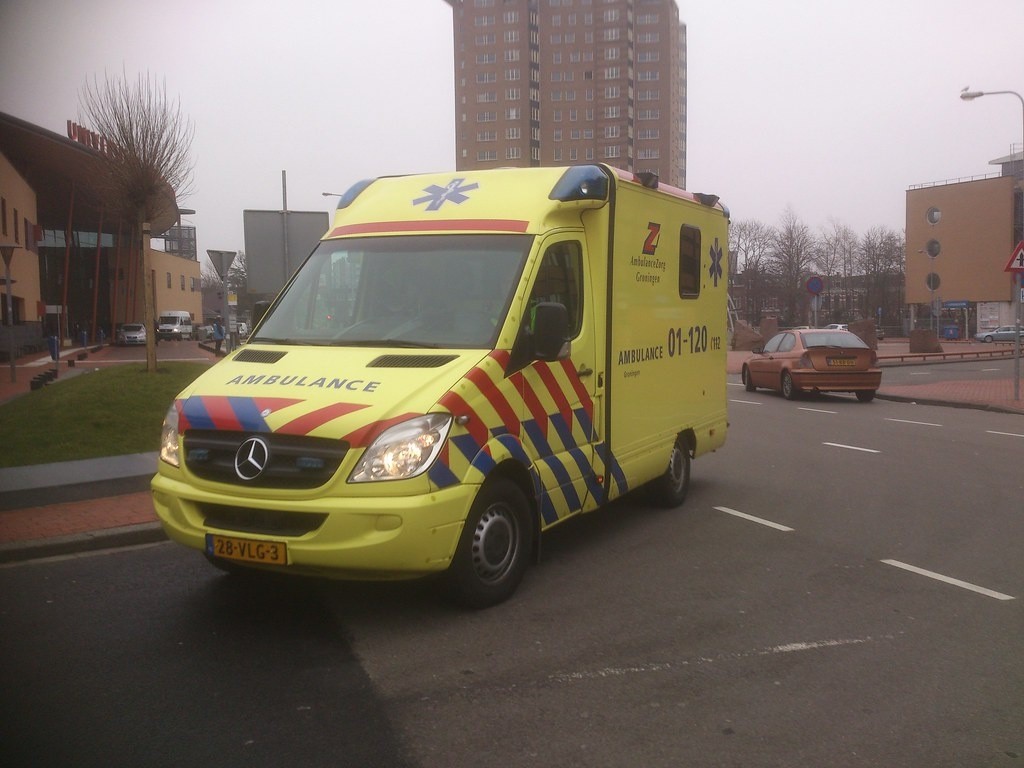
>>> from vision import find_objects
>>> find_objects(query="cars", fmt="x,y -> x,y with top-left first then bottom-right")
115,322 -> 148,346
742,329 -> 883,401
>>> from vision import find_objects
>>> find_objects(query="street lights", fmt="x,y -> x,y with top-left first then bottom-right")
320,189 -> 343,202
960,85 -> 1023,148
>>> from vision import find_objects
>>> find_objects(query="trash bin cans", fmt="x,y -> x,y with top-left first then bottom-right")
944,325 -> 959,341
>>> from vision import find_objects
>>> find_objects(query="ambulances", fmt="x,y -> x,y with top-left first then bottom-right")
153,161 -> 732,608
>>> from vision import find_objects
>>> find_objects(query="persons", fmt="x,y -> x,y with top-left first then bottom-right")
213,315 -> 224,357
486,273 -> 538,335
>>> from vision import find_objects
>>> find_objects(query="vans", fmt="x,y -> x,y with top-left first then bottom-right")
158,309 -> 193,342
